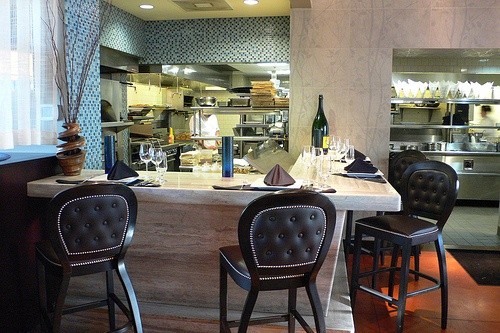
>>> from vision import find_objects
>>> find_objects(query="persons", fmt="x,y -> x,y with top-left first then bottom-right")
477,105 -> 497,138
189,110 -> 222,154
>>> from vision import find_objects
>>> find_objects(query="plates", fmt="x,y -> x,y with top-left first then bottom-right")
341,165 -> 384,177
363,156 -> 371,162
88,174 -> 144,181
250,177 -> 304,189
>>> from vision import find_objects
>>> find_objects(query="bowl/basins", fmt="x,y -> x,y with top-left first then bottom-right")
415,103 -> 439,107
128,105 -> 153,116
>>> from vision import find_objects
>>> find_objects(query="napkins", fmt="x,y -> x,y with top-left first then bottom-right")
107,159 -> 139,179
263,163 -> 296,187
347,148 -> 366,159
343,157 -> 378,173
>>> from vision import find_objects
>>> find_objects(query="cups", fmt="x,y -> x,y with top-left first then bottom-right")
104,135 -> 115,174
222,136 -> 234,178
345,144 -> 355,164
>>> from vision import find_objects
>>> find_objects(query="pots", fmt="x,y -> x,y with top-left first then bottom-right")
196,96 -> 216,106
389,142 -> 441,150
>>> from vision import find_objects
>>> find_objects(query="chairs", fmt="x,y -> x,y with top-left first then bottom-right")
349,149 -> 459,332
217,189 -> 336,333
33,183 -> 143,333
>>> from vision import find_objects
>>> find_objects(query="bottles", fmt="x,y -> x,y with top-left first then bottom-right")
311,95 -> 329,156
168,127 -> 174,144
469,129 -> 475,143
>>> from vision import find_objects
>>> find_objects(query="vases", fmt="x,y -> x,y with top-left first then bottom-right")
56,121 -> 86,177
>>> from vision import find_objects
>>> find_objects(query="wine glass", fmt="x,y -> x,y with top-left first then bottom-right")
302,146 -> 331,188
335,137 -> 349,173
326,134 -> 341,172
139,142 -> 168,183
